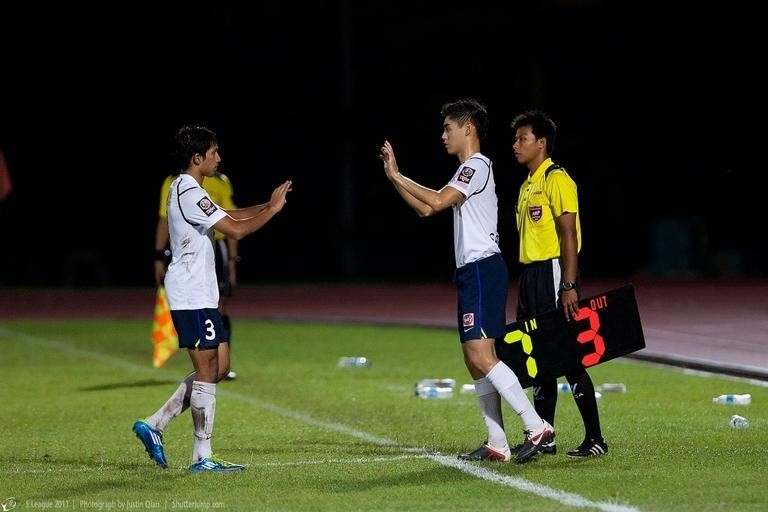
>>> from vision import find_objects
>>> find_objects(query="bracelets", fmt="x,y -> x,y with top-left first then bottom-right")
154,248 -> 165,263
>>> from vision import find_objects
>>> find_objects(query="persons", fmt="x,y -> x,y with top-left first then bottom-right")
379,100 -> 554,463
133,125 -> 294,471
508,111 -> 608,455
153,125 -> 237,379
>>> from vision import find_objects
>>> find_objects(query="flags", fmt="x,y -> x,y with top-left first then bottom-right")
152,285 -> 179,370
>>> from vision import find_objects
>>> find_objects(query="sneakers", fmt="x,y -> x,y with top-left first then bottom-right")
458,440 -> 511,463
188,455 -> 245,472
510,438 -> 558,458
133,421 -> 168,469
565,440 -> 610,458
516,417 -> 556,463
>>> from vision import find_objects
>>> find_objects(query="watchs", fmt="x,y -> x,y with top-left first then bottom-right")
559,281 -> 576,290
229,254 -> 241,263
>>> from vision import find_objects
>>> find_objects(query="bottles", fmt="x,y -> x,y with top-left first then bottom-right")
337,356 -> 369,369
711,393 -> 752,406
728,413 -> 750,431
414,377 -> 626,400
